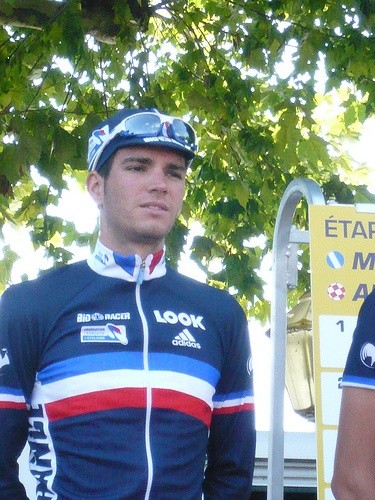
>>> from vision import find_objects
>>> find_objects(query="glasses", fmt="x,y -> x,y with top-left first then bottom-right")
90,112 -> 198,173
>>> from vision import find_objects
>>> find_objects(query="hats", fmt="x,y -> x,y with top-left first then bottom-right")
87,108 -> 198,173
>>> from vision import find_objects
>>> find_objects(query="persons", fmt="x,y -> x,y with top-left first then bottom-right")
330,285 -> 375,500
1,110 -> 257,500
